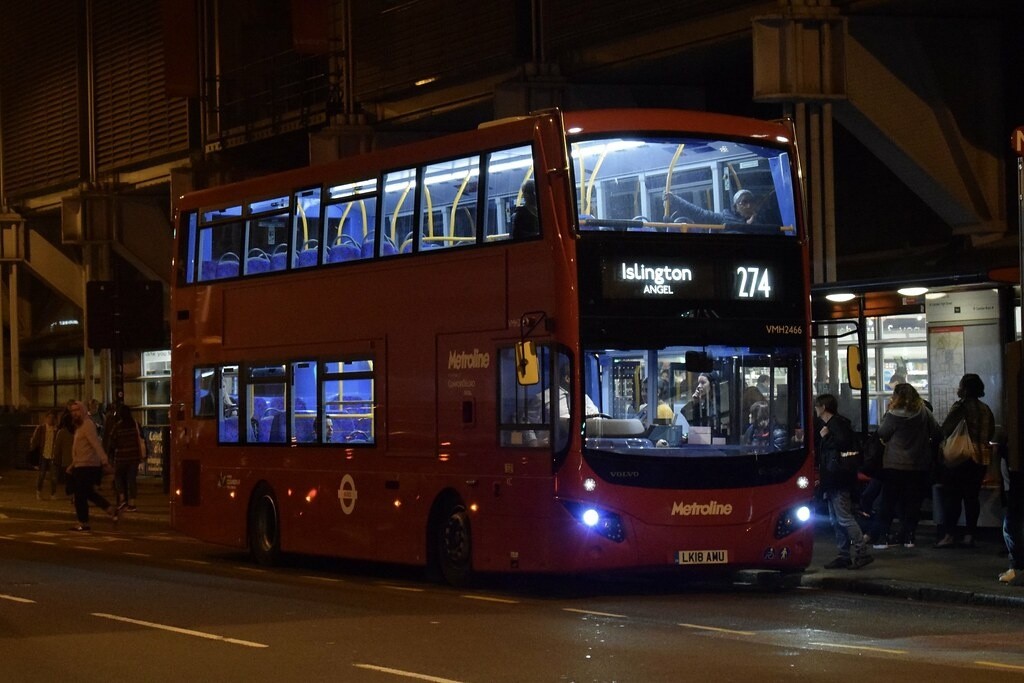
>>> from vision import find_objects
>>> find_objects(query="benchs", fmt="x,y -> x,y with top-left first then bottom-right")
224,396 -> 372,442
214,217 -> 699,280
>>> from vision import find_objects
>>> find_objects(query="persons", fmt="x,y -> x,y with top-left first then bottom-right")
0,398 -> 148,532
640,368 -> 1024,586
666,191 -> 772,234
510,180 -> 541,237
520,362 -> 599,449
199,382 -> 333,442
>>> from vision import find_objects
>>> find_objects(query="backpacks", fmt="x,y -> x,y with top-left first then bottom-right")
863,426 -> 885,465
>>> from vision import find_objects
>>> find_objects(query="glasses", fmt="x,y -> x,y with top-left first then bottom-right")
736,200 -> 754,204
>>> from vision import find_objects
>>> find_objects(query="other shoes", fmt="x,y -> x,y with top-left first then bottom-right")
955,541 -> 974,549
111,508 -> 121,524
824,555 -> 852,569
932,541 -> 955,549
903,537 -> 915,548
872,538 -> 889,550
998,569 -> 1024,583
847,556 -> 873,570
51,495 -> 61,500
117,502 -> 129,513
125,505 -> 136,512
36,490 -> 41,500
68,525 -> 92,536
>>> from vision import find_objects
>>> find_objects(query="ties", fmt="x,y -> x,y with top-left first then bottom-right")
566,392 -> 571,415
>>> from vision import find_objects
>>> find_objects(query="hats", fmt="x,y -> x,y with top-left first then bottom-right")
733,190 -> 755,204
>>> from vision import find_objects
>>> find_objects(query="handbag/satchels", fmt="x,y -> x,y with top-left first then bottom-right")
827,416 -> 866,472
136,422 -> 147,458
812,483 -> 829,516
939,401 -> 976,468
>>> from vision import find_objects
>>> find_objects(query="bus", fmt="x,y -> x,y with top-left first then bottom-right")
171,106 -> 862,589
